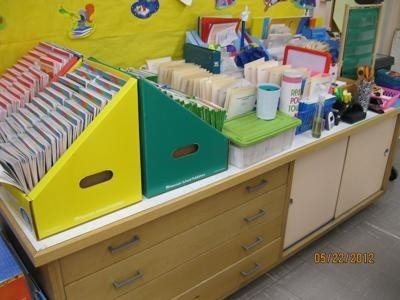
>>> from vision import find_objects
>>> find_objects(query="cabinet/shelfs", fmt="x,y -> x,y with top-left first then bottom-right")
46,153 -> 295,300
281,109 -> 400,251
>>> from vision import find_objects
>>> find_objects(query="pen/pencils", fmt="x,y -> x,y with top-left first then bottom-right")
334,87 -> 352,103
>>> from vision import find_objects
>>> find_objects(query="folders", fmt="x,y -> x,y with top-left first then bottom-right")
184,43 -> 220,73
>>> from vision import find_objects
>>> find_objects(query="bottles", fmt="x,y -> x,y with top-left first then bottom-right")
311,95 -> 326,138
279,69 -> 303,117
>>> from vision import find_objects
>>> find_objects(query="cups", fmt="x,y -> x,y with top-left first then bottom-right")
255,83 -> 281,120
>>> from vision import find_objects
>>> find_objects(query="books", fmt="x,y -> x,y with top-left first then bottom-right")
0,39 -> 129,197
119,17 -> 333,134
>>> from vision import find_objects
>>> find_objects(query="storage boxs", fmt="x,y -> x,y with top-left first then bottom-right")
223,109 -> 302,169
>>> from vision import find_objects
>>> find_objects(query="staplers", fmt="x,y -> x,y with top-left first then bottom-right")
368,97 -> 384,114
